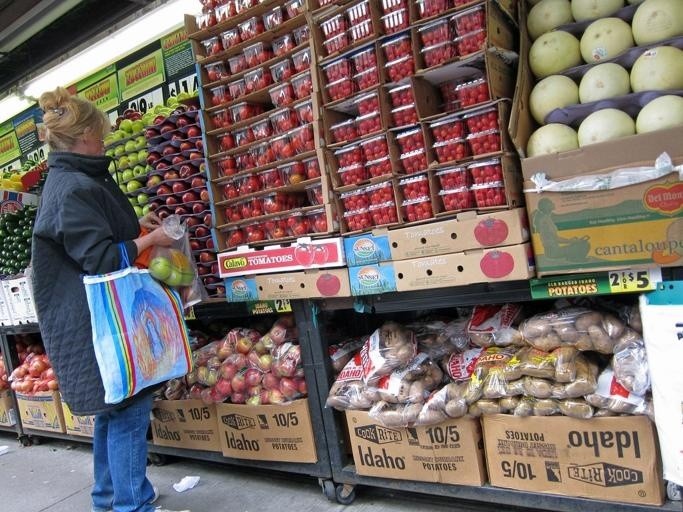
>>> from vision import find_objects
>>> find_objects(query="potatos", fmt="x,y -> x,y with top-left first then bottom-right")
317,299 -> 653,428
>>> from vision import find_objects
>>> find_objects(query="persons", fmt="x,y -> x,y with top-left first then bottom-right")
32,86 -> 190,512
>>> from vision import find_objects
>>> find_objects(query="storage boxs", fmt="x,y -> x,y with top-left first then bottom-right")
0,278 -> 13,326
59,392 -> 95,439
150,398 -> 222,452
216,398 -> 318,464
2,266 -> 38,326
346,409 -> 486,487
480,413 -> 666,507
16,390 -> 66,434
0,388 -> 16,427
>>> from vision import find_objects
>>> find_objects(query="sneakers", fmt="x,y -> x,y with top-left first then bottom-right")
153,505 -> 190,512
148,486 -> 158,504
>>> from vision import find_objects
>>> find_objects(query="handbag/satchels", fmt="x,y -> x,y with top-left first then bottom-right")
82,266 -> 195,405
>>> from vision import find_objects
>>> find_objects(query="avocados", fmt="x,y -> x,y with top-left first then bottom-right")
0,204 -> 38,278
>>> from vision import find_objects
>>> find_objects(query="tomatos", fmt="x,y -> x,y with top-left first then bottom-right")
318,2 -> 510,233
480,250 -> 514,278
294,243 -> 314,267
196,0 -> 331,249
313,245 -> 329,264
316,272 -> 340,296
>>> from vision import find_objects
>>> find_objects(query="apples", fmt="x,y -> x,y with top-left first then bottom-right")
0,344 -> 59,391
165,325 -> 307,407
96,88 -> 224,296
